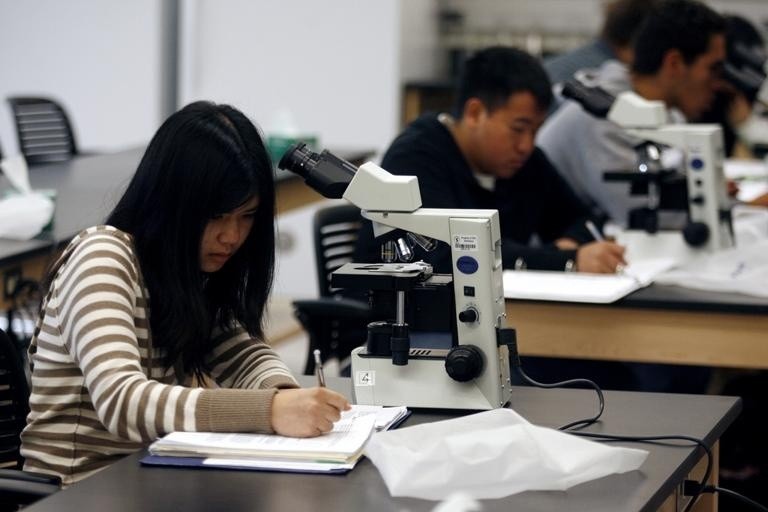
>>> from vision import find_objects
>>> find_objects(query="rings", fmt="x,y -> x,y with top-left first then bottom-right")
583,220 -> 604,243
313,348 -> 326,387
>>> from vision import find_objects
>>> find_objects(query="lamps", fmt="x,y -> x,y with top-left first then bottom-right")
0,328 -> 62,512
7,94 -> 80,169
301,201 -> 376,376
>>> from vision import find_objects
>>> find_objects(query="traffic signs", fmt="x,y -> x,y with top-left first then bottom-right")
676,462 -> 702,512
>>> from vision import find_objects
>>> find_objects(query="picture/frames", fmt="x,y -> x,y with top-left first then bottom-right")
486,250 -> 768,376
11,376 -> 739,512
0,146 -> 377,313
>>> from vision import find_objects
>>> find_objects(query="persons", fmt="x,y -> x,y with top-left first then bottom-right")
379,44 -> 622,284
536,0 -> 766,227
21,103 -> 356,493
